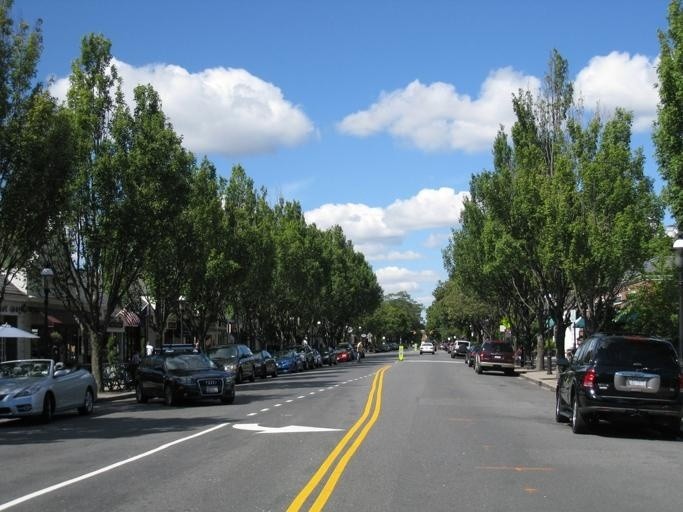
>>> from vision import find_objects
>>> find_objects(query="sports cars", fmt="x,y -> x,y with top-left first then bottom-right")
0,358 -> 98,418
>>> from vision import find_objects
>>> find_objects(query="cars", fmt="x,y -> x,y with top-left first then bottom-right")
464,345 -> 480,367
392,342 -> 397,349
135,352 -> 235,406
380,344 -> 391,351
252,350 -> 278,379
311,349 -> 337,367
271,351 -> 304,373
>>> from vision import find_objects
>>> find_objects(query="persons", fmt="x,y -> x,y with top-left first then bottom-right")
356,339 -> 364,363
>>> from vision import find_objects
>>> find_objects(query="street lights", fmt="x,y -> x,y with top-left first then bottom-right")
398,330 -> 415,344
670,239 -> 682,358
316,320 -> 321,349
176,294 -> 190,344
469,324 -> 472,337
543,293 -> 552,374
39,261 -> 53,359
288,314 -> 295,345
447,335 -> 456,342
358,326 -> 361,341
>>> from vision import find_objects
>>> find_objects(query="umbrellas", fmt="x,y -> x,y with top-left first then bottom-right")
0,319 -> 42,340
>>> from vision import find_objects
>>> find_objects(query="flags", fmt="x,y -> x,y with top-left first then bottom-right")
117,299 -> 144,325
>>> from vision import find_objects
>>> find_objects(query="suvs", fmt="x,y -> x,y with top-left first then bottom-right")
334,342 -> 354,362
419,343 -> 435,354
206,343 -> 255,382
447,343 -> 454,354
451,340 -> 470,358
551,331 -> 682,432
475,340 -> 514,376
440,343 -> 445,350
283,344 -> 313,368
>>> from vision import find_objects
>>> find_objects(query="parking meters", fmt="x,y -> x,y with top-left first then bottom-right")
356,342 -> 363,363
